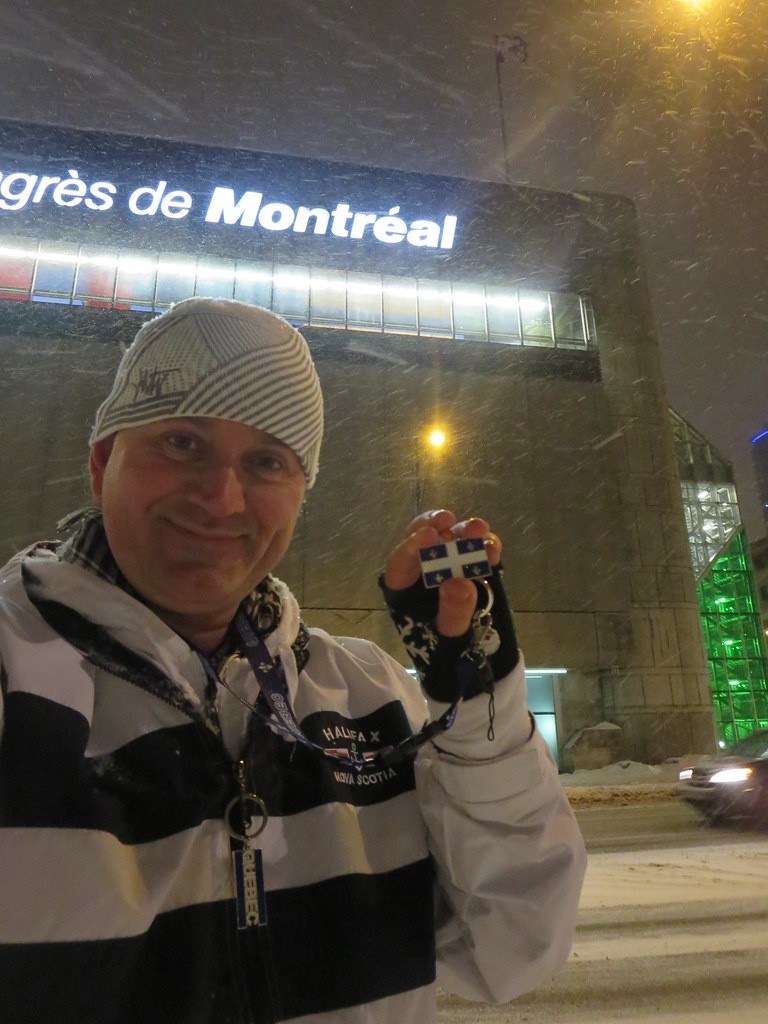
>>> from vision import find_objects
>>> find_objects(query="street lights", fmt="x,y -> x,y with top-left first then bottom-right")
413,426 -> 450,519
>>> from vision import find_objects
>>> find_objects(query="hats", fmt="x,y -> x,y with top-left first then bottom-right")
88,297 -> 323,488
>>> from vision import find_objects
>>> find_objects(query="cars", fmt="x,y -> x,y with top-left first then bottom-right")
677,728 -> 768,829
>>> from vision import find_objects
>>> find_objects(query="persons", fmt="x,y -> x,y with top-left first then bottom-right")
0,297 -> 586,1024
